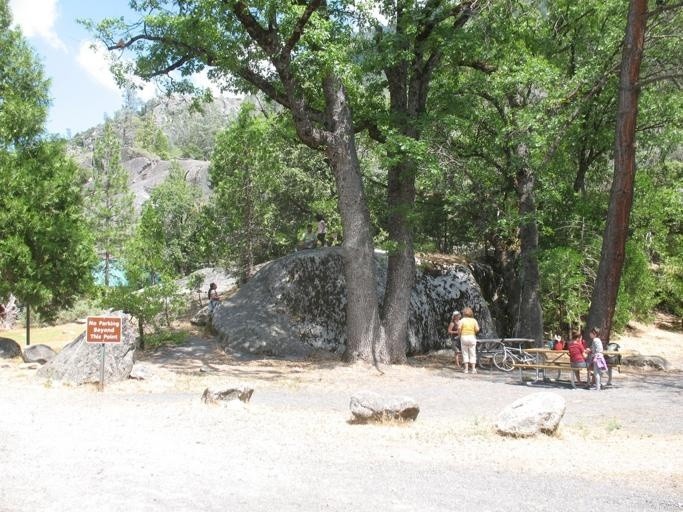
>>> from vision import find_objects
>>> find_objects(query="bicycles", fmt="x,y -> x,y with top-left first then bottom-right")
453,336 -> 544,371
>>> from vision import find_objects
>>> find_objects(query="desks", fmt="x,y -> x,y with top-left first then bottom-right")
521,347 -> 631,390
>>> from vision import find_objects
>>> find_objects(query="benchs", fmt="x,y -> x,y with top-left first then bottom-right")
512,360 -> 621,389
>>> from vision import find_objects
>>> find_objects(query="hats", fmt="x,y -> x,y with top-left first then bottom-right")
451,311 -> 461,318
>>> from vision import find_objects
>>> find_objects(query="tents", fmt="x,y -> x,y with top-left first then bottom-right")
92,258 -> 129,287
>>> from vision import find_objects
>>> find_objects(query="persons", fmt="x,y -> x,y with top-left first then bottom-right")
317,215 -> 326,247
208,283 -> 220,317
448,310 -> 461,368
304,224 -> 318,249
546,327 -> 608,390
457,307 -> 479,374
